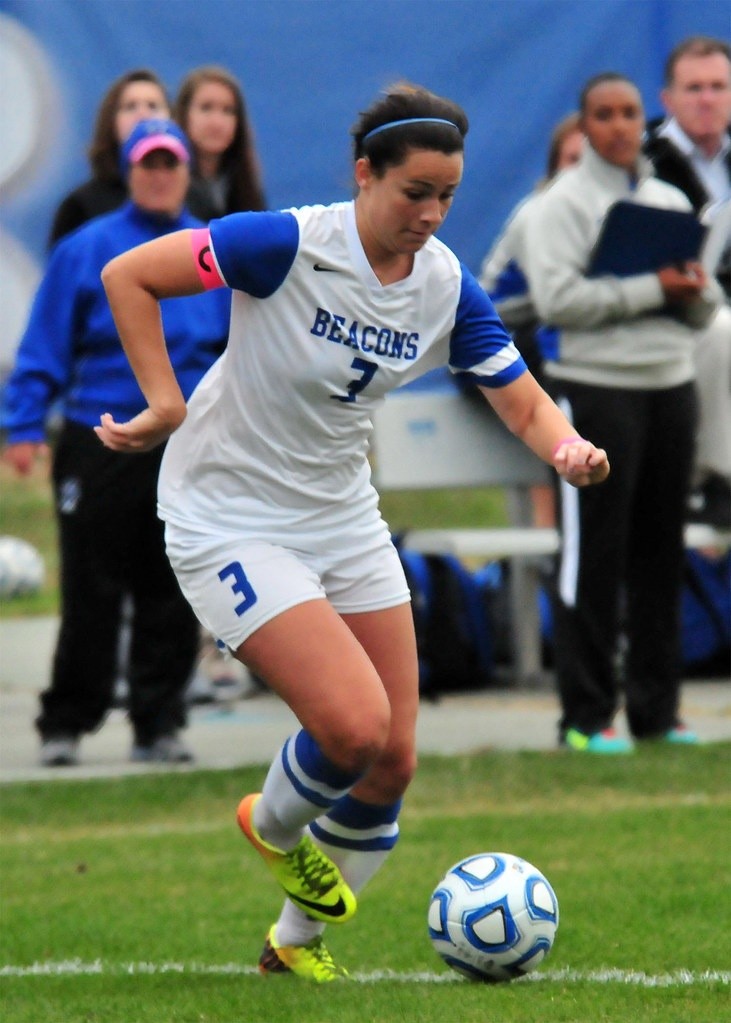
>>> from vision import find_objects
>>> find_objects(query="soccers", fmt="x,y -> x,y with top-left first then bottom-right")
426,849 -> 560,983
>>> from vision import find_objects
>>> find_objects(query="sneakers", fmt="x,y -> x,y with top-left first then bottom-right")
129,734 -> 192,768
256,927 -> 352,984
36,735 -> 81,770
624,723 -> 714,747
234,793 -> 358,927
560,725 -> 635,757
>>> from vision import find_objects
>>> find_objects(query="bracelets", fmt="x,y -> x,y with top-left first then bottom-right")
553,438 -> 585,458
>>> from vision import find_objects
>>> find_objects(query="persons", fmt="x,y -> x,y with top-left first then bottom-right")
41,71 -> 172,703
179,67 -> 266,700
93,88 -> 610,983
477,40 -> 731,752
1,121 -> 233,767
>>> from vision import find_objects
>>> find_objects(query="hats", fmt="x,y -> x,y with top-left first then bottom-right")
124,119 -> 189,172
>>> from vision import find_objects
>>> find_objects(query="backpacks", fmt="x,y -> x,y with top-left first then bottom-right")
388,535 -> 730,699
